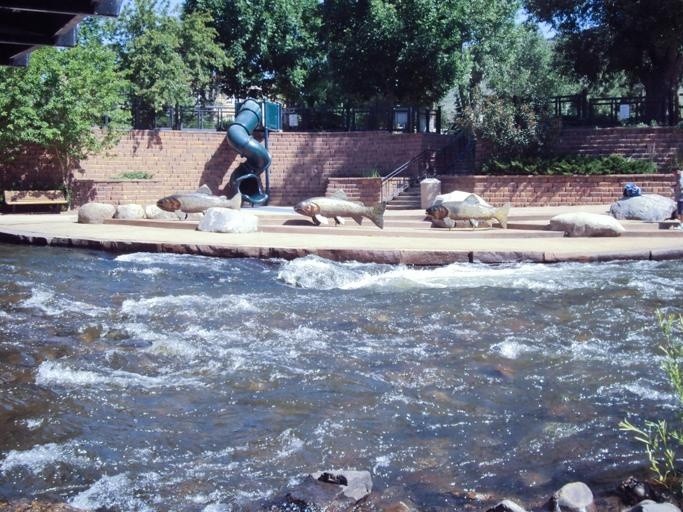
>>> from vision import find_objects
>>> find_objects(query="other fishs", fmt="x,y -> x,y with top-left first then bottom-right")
425,194 -> 510,230
158,183 -> 242,222
294,189 -> 386,230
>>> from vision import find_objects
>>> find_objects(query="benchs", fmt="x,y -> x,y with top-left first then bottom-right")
3,190 -> 67,214
